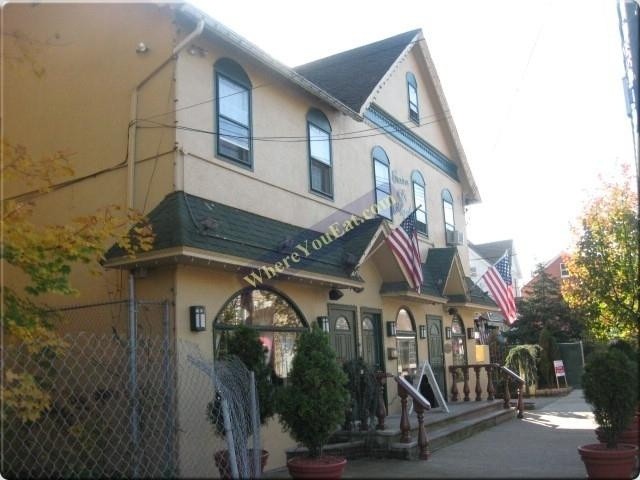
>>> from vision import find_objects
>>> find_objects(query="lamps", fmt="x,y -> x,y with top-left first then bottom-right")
420,325 -> 427,338
387,321 -> 397,336
329,288 -> 343,301
317,315 -> 330,333
190,306 -> 206,332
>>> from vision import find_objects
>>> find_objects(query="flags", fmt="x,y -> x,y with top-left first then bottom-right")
482,252 -> 517,326
385,211 -> 424,295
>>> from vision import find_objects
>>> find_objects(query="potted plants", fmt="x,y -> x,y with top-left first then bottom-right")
574,336 -> 639,478
204,319 -> 354,479
